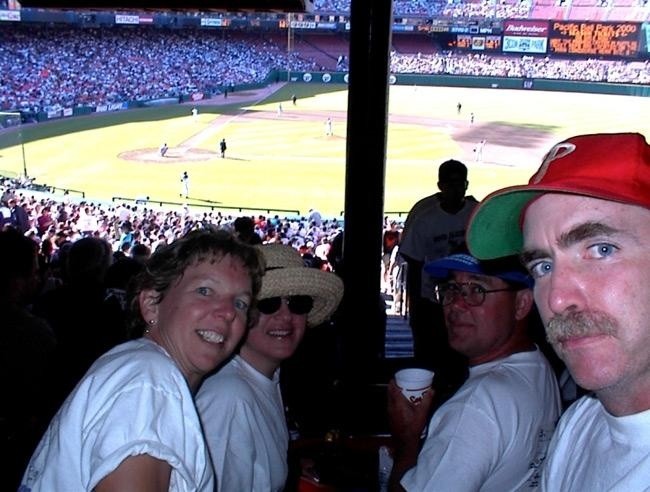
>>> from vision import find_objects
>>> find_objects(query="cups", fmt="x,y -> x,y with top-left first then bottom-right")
393,367 -> 433,440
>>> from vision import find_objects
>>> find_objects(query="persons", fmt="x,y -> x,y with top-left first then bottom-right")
469,113 -> 475,124
385,246 -> 564,492
323,117 -> 333,136
0,173 -> 408,492
220,138 -> 226,158
313,0 -> 649,85
160,143 -> 169,157
2,0 -> 326,113
400,158 -> 484,370
474,139 -> 487,161
464,128 -> 649,492
192,106 -> 198,123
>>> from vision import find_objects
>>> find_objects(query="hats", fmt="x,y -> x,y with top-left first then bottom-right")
465,132 -> 650,260
254,241 -> 344,329
422,252 -> 533,284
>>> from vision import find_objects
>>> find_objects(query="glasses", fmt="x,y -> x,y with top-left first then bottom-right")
258,292 -> 313,314
432,280 -> 508,307
